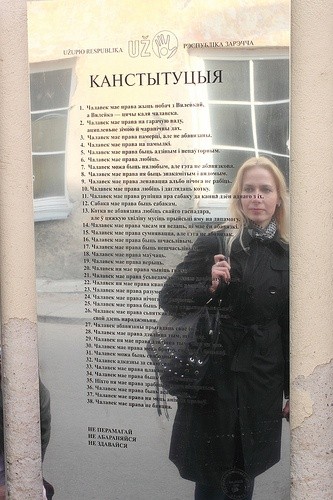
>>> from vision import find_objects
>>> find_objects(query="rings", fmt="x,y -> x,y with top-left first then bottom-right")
215,262 -> 220,267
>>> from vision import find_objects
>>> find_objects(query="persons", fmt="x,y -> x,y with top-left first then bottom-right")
155,158 -> 291,498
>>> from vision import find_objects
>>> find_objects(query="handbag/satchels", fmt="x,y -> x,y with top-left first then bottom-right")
145,228 -> 227,399
